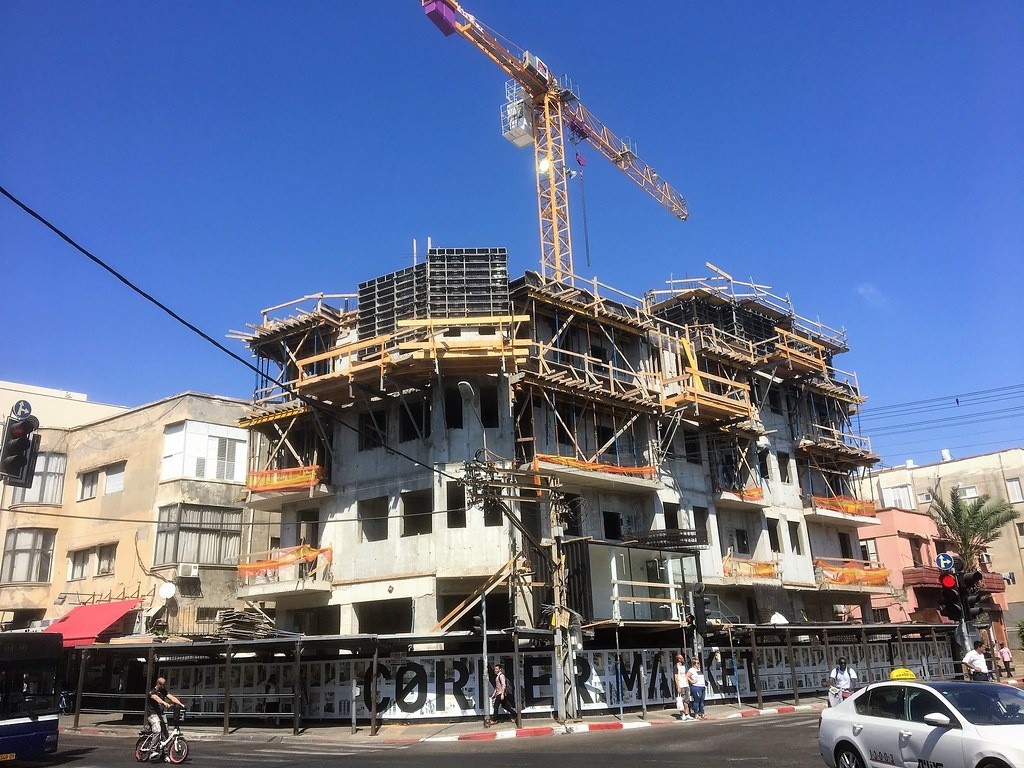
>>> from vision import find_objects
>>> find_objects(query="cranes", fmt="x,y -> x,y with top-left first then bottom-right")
415,0 -> 689,286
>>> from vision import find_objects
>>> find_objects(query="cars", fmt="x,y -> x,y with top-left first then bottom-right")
818,667 -> 1024,768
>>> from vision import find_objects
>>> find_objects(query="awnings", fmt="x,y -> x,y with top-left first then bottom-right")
42,597 -> 144,647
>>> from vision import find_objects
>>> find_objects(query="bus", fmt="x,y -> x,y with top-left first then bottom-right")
0,630 -> 64,763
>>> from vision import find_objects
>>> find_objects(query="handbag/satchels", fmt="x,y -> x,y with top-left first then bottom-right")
676,692 -> 684,712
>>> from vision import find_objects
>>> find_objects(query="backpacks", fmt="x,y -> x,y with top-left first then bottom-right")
499,673 -> 513,696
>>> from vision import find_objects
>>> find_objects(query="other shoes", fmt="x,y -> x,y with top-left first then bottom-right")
490,720 -> 499,724
163,754 -> 171,762
149,751 -> 159,759
681,713 -> 686,720
702,714 -> 707,719
695,714 -> 701,719
686,714 -> 693,719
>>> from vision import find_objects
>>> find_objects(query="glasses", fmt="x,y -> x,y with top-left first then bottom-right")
840,662 -> 846,665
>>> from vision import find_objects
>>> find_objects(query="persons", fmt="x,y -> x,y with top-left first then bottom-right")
673,654 -> 708,721
821,657 -> 857,708
146,677 -> 186,762
490,664 -> 517,725
998,641 -> 1013,678
961,640 -> 989,681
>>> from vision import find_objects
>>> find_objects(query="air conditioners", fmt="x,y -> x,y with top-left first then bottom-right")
980,553 -> 993,564
176,563 -> 200,579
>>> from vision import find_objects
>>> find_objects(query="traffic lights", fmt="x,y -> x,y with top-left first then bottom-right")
694,597 -> 712,634
0,416 -> 35,479
685,614 -> 695,624
938,572 -> 963,621
957,572 -> 984,622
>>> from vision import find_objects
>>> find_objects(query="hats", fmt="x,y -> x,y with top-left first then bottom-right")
1000,642 -> 1004,645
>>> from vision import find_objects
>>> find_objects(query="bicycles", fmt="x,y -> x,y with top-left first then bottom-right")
135,705 -> 189,764
